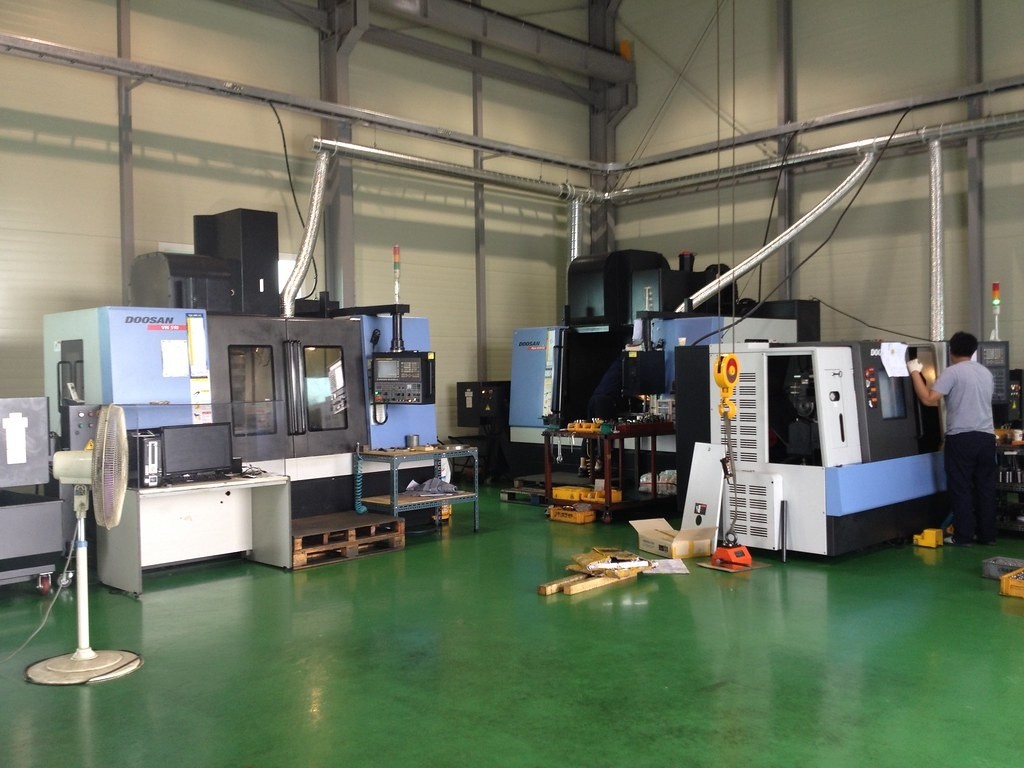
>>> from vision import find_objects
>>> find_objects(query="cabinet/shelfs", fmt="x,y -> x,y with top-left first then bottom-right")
994,443 -> 1024,532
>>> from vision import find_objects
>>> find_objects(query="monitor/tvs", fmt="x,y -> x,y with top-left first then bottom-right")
158,421 -> 234,475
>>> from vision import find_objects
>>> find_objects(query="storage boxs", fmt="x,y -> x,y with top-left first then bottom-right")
994,429 -> 1024,446
548,507 -> 596,524
551,486 -> 591,501
579,490 -> 622,503
982,556 -> 1024,579
998,567 -> 1024,598
627,518 -> 720,561
567,423 -> 604,433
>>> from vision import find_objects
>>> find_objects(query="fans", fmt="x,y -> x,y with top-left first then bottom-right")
23,402 -> 142,687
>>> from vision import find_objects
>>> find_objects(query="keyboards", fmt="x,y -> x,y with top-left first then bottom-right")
160,473 -> 232,487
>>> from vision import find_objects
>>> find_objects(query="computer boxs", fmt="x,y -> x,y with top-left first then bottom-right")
126,430 -> 163,489
211,207 -> 282,317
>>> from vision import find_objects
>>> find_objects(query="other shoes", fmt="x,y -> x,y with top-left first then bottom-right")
944,537 -> 976,547
973,533 -> 995,546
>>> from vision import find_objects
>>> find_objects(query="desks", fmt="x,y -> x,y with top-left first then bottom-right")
541,425 -> 675,523
356,445 -> 479,536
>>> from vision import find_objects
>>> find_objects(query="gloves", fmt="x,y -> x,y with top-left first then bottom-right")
907,359 -> 924,374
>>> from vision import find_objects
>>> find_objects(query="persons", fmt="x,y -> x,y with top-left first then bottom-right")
908,330 -> 999,546
